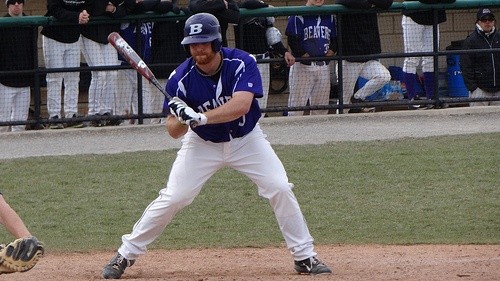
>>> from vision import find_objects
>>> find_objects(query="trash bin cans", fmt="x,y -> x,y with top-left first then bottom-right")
445,40 -> 469,98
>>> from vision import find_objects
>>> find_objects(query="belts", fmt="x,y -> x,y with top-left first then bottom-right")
299,60 -> 330,66
255,53 -> 269,59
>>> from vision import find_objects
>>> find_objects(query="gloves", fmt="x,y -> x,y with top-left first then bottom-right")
167,96 -> 207,126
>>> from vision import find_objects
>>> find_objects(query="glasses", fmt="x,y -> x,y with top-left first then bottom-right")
479,18 -> 494,22
8,0 -> 23,5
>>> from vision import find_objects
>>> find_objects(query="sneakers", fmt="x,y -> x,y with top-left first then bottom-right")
293,256 -> 333,274
102,252 -> 135,280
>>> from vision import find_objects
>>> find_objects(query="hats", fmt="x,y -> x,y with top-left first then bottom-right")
477,8 -> 495,19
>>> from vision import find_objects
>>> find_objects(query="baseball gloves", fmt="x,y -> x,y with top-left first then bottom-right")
0,237 -> 44,275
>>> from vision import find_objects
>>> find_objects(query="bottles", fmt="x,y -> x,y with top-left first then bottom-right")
382,81 -> 404,99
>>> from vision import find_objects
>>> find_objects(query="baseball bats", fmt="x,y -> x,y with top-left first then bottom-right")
108,33 -> 198,129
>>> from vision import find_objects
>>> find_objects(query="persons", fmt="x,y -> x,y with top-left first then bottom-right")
232,0 -> 295,117
336,0 -> 393,114
40,0 -> 90,129
103,12 -> 332,279
0,0 -> 38,132
0,191 -> 45,274
459,8 -> 500,107
80,0 -> 242,127
285,0 -> 338,117
401,0 -> 456,108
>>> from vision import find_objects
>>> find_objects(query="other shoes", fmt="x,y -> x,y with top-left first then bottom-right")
408,97 -> 426,107
350,96 -> 376,112
89,114 -> 125,126
66,119 -> 84,128
46,122 -> 65,129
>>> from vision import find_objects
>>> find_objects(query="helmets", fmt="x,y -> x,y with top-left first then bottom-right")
181,12 -> 221,46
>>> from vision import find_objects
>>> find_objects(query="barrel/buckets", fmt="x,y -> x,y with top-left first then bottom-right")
424,72 -> 447,97
447,40 -> 470,97
